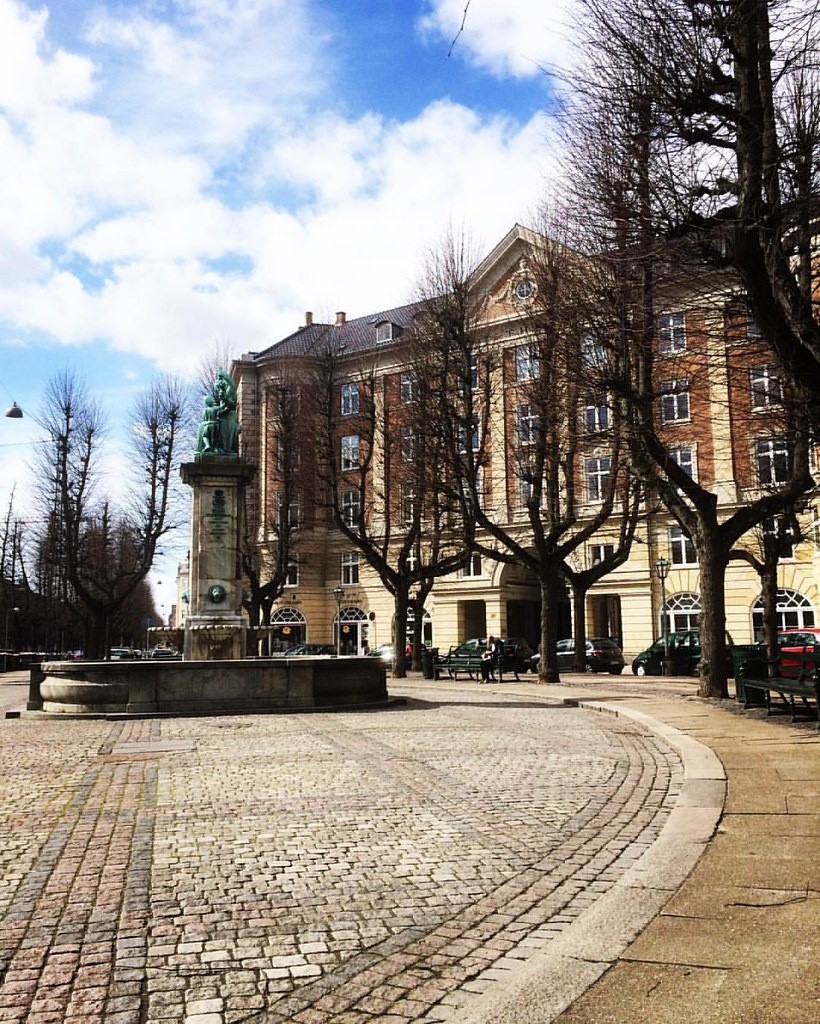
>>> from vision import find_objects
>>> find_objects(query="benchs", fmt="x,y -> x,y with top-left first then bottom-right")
431,644 -> 524,685
739,639 -> 820,730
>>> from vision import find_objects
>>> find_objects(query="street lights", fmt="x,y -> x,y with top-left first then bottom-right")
332,586 -> 345,657
654,555 -> 676,678
3,606 -> 21,674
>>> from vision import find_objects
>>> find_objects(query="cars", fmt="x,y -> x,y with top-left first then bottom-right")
102,645 -> 180,662
777,628 -> 820,680
529,638 -> 624,675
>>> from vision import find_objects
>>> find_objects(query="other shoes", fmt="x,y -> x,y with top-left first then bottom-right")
478,679 -> 483,684
483,679 -> 488,683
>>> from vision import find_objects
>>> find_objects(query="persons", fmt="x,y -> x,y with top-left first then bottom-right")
478,636 -> 504,684
405,641 -> 412,671
66,653 -> 72,661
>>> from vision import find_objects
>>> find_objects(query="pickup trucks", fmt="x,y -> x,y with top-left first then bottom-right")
631,630 -> 767,679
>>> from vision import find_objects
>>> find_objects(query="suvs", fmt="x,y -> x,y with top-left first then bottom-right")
283,643 -> 334,655
450,636 -> 535,674
366,642 -> 430,672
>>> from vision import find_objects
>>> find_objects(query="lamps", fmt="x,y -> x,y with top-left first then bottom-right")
5,401 -> 22,419
